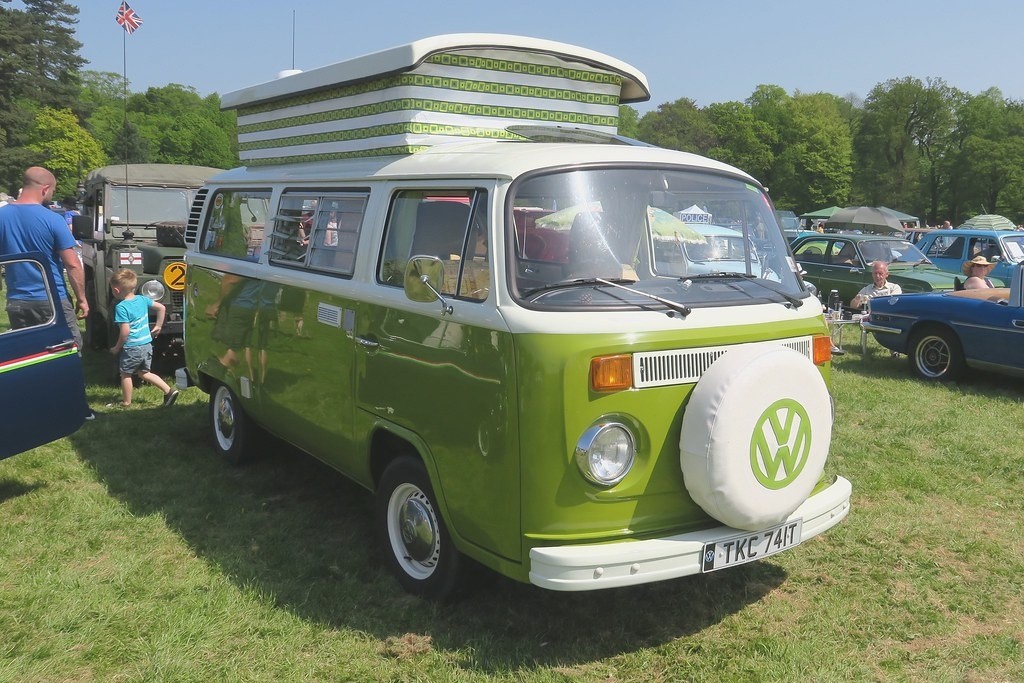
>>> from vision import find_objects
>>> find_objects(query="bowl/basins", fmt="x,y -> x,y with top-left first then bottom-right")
861,311 -> 867,315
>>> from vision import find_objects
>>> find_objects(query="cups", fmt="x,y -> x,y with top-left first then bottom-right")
860,295 -> 868,304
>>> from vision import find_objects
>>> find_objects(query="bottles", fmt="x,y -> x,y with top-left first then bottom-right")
828,289 -> 839,318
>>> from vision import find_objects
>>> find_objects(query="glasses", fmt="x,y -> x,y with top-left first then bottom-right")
976,264 -> 988,268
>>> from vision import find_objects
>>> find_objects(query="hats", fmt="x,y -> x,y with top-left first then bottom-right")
796,262 -> 807,275
962,256 -> 996,276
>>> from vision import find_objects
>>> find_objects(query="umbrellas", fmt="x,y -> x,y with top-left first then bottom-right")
958,214 -> 1017,231
826,204 -> 905,234
535,199 -> 705,246
799,206 -> 845,219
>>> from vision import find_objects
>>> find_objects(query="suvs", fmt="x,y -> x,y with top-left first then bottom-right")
72,163 -> 230,384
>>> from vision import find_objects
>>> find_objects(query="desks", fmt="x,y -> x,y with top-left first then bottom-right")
824,318 -> 868,355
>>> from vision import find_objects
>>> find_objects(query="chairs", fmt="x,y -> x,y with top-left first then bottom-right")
412,200 -> 476,262
570,212 -> 633,278
953,277 -> 965,290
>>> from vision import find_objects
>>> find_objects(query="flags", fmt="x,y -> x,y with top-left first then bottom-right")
116,1 -> 144,35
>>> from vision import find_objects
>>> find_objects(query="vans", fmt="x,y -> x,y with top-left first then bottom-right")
653,224 -> 762,277
182,31 -> 854,596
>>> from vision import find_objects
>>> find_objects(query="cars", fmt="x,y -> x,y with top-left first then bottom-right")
0,252 -> 89,461
789,234 -> 1005,319
860,260 -> 1024,382
712,224 -> 776,260
888,228 -> 938,245
889,230 -> 1024,276
784,230 -> 821,245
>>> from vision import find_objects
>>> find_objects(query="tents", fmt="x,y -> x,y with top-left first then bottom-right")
878,206 -> 920,227
673,204 -> 713,224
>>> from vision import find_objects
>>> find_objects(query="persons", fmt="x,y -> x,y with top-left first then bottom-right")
108,268 -> 179,409
941,221 -> 953,247
850,261 -> 902,358
962,256 -> 1004,304
203,257 -> 306,389
752,217 -> 764,238
0,192 -> 8,207
816,222 -> 825,233
299,199 -> 338,246
0,166 -> 96,422
766,256 -> 844,355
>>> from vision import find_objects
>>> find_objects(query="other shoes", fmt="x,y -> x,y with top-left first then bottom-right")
105,400 -> 131,408
831,347 -> 843,355
163,388 -> 180,406
84,411 -> 97,420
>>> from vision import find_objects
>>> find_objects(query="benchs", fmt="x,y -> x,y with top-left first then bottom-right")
946,288 -> 1011,304
